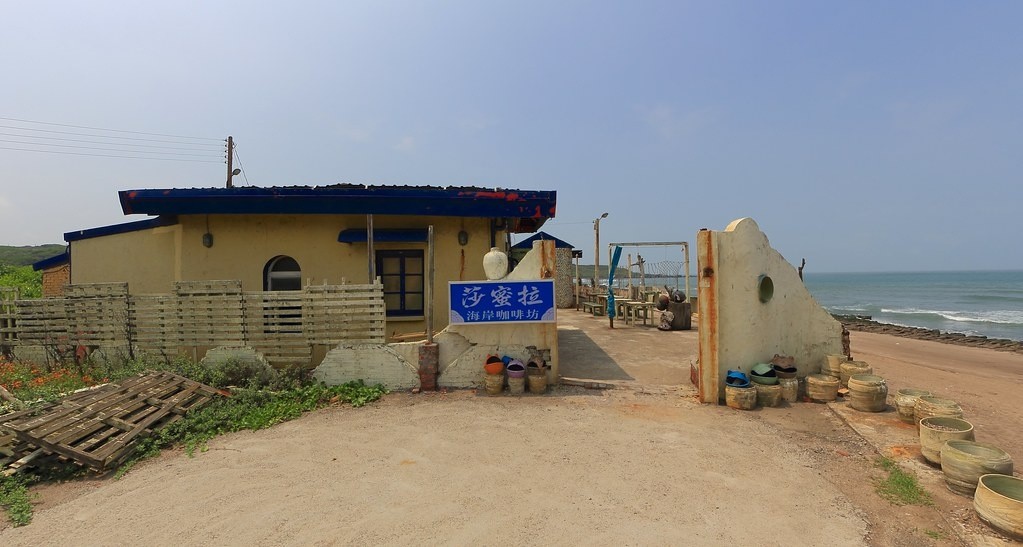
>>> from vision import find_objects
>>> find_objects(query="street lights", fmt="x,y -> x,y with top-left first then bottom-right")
593,213 -> 608,294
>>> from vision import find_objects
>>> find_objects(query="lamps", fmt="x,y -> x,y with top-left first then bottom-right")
203,233 -> 213,248
458,230 -> 467,246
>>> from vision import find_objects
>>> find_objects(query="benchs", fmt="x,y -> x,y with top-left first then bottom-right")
582,302 -> 603,316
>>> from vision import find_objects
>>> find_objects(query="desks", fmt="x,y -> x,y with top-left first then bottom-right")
587,293 -> 640,320
624,302 -> 656,327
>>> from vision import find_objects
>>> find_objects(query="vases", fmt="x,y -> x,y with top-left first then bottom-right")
724,354 -> 798,409
804,353 -> 888,412
895,389 -> 1023,540
484,355 -> 548,395
